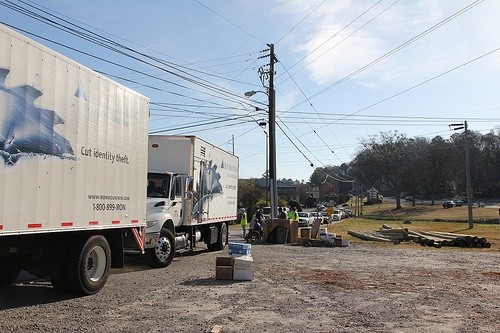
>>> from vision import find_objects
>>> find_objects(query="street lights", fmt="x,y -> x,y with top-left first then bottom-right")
244,89 -> 277,217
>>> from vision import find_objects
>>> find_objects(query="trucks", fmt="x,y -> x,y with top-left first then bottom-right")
124,135 -> 239,269
0,22 -> 148,297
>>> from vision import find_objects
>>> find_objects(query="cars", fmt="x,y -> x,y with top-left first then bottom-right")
316,201 -> 352,224
442,199 -> 463,209
404,195 -> 413,201
311,212 -> 324,225
298,211 -> 315,227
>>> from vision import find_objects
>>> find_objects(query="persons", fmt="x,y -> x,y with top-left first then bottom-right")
278,206 -> 299,222
249,206 -> 263,232
241,208 -> 247,236
155,178 -> 167,193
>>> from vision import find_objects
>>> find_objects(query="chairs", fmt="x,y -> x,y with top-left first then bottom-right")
312,213 -> 315,216
298,214 -> 302,217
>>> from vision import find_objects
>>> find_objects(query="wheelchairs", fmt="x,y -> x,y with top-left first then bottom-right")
247,226 -> 266,244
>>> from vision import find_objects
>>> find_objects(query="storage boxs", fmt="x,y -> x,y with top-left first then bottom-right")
233,269 -> 253,281
215,256 -> 232,266
228,242 -> 252,255
232,253 -> 254,269
215,265 -> 233,280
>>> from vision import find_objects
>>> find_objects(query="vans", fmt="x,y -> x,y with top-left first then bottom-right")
261,206 -> 289,220
